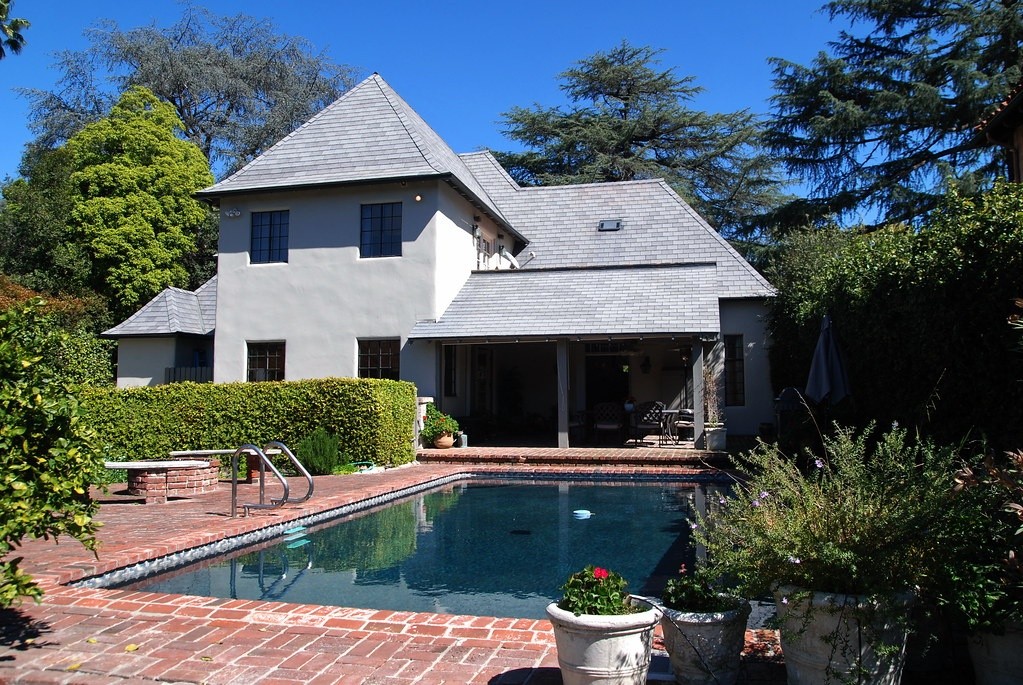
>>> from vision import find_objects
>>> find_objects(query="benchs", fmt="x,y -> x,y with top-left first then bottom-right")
169,449 -> 282,486
104,460 -> 210,502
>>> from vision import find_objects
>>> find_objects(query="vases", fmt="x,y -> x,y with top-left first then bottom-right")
434,431 -> 455,449
545,593 -> 664,685
768,580 -> 918,685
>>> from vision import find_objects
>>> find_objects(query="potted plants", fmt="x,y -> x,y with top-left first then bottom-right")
661,576 -> 753,685
704,415 -> 727,450
947,449 -> 1023,685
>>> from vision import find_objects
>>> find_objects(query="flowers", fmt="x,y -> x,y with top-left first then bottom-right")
558,564 -> 632,616
418,403 -> 459,441
684,419 -> 1013,685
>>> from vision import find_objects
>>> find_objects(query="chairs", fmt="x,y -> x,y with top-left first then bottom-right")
570,400 -> 694,448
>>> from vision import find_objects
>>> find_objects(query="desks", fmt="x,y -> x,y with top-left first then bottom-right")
577,410 -> 680,445
